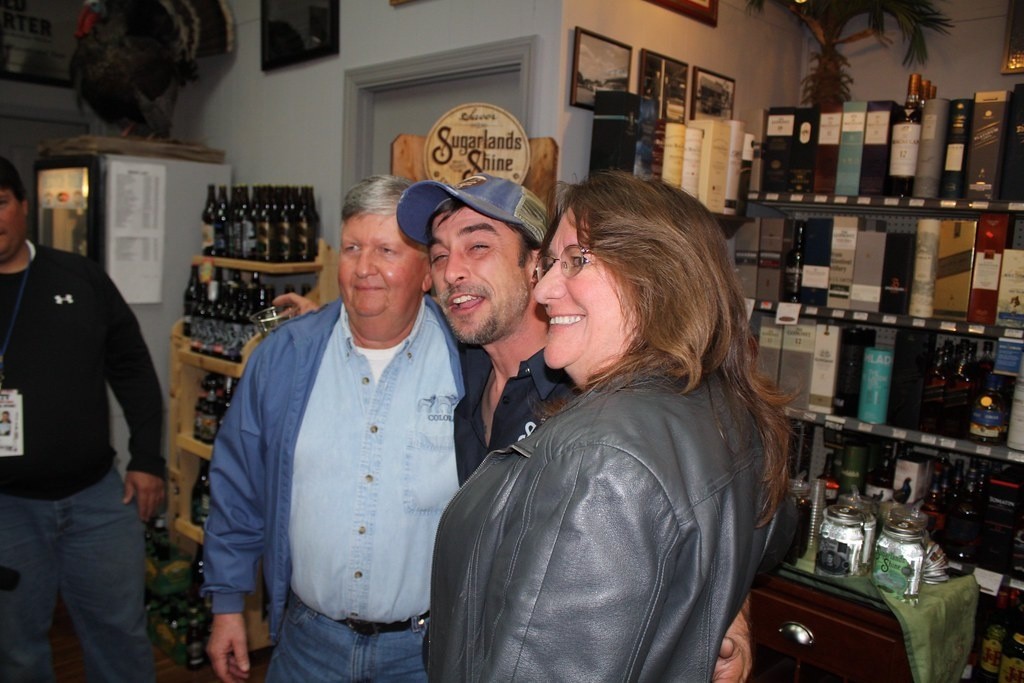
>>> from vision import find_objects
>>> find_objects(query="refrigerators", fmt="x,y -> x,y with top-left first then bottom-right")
32,156 -> 233,536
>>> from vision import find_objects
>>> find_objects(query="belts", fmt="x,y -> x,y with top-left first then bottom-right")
336,611 -> 429,634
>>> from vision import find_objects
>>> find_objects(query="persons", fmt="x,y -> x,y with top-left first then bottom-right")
201,177 -> 470,683
0,152 -> 169,683
272,174 -> 751,683
421,168 -> 793,683
0,412 -> 11,435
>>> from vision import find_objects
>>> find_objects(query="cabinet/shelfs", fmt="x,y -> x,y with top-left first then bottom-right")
717,191 -> 1024,683
170,238 -> 326,650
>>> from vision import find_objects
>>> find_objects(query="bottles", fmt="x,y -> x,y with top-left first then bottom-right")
916,338 -> 1014,445
783,220 -> 805,304
183,265 -> 312,364
886,69 -> 936,199
957,586 -> 1024,683
138,374 -> 240,670
201,182 -> 319,263
776,435 -> 1003,594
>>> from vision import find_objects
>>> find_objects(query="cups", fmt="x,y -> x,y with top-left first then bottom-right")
249,304 -> 300,340
857,346 -> 894,427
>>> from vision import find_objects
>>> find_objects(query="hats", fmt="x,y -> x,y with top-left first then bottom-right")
396,173 -> 552,245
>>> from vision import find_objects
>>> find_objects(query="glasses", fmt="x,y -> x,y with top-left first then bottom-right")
535,245 -> 594,278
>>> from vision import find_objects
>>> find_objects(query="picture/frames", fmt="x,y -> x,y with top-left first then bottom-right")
1000,0 -> 1024,74
567,0 -> 736,126
260,0 -> 339,70
